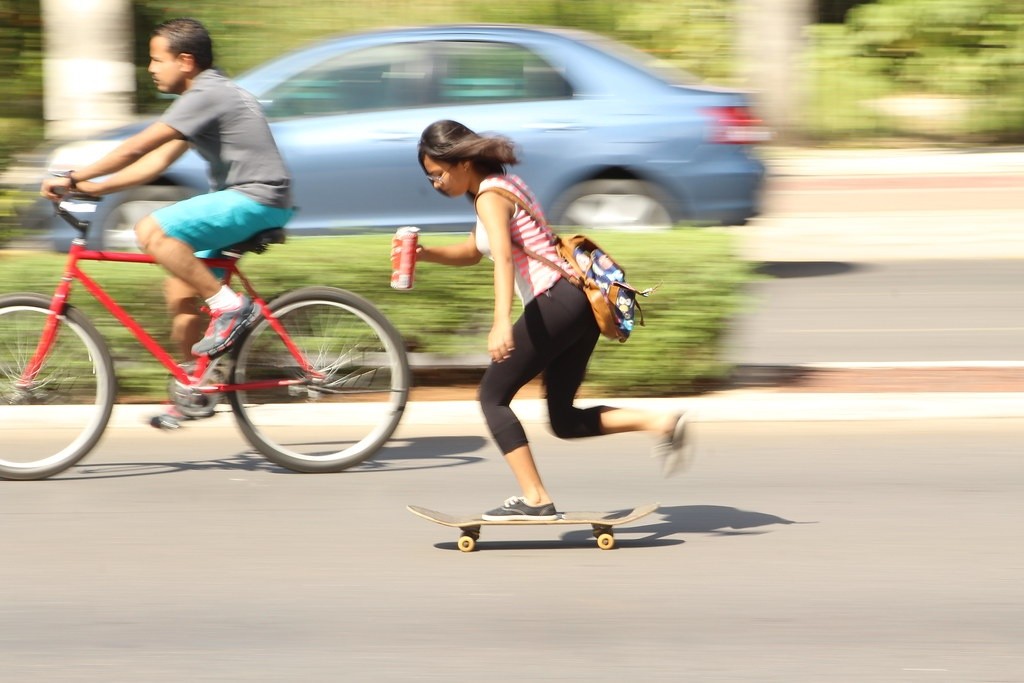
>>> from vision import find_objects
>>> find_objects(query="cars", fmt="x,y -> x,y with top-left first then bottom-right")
13,19 -> 768,233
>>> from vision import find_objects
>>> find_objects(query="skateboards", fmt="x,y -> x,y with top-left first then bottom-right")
407,501 -> 661,551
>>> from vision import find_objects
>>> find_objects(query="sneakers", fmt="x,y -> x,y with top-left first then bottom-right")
482,496 -> 560,522
150,396 -> 216,430
190,289 -> 262,358
660,411 -> 689,480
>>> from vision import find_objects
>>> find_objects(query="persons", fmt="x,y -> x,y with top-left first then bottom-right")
41,16 -> 297,432
389,119 -> 690,522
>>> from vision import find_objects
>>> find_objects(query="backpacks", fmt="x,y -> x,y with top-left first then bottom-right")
473,187 -> 651,344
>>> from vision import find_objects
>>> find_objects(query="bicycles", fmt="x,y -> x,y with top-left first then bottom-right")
0,188 -> 410,483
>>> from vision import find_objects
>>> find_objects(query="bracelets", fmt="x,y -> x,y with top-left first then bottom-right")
51,169 -> 79,190
